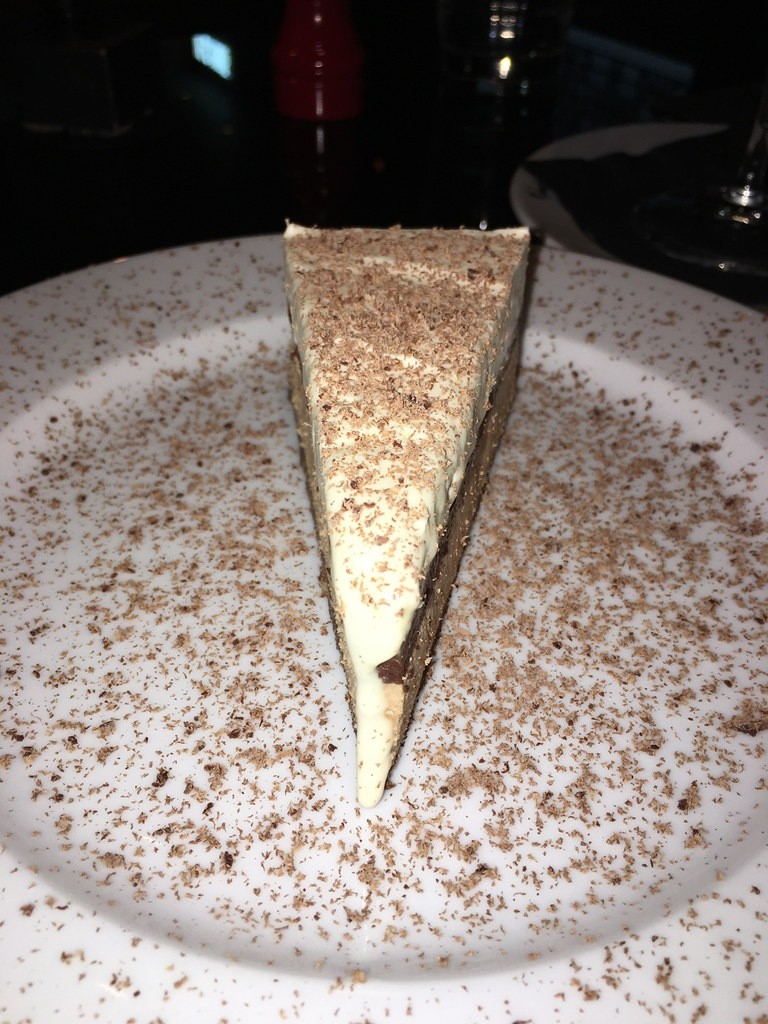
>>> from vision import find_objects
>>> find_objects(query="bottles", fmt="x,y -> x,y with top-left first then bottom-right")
464,1 -> 545,235
274,1 -> 364,230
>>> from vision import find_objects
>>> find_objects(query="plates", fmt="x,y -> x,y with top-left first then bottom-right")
512,122 -> 768,318
0,239 -> 768,1024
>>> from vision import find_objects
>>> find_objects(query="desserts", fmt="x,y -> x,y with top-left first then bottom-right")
284,222 -> 532,806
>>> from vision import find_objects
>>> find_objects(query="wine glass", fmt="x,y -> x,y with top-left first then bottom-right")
634,83 -> 768,276
